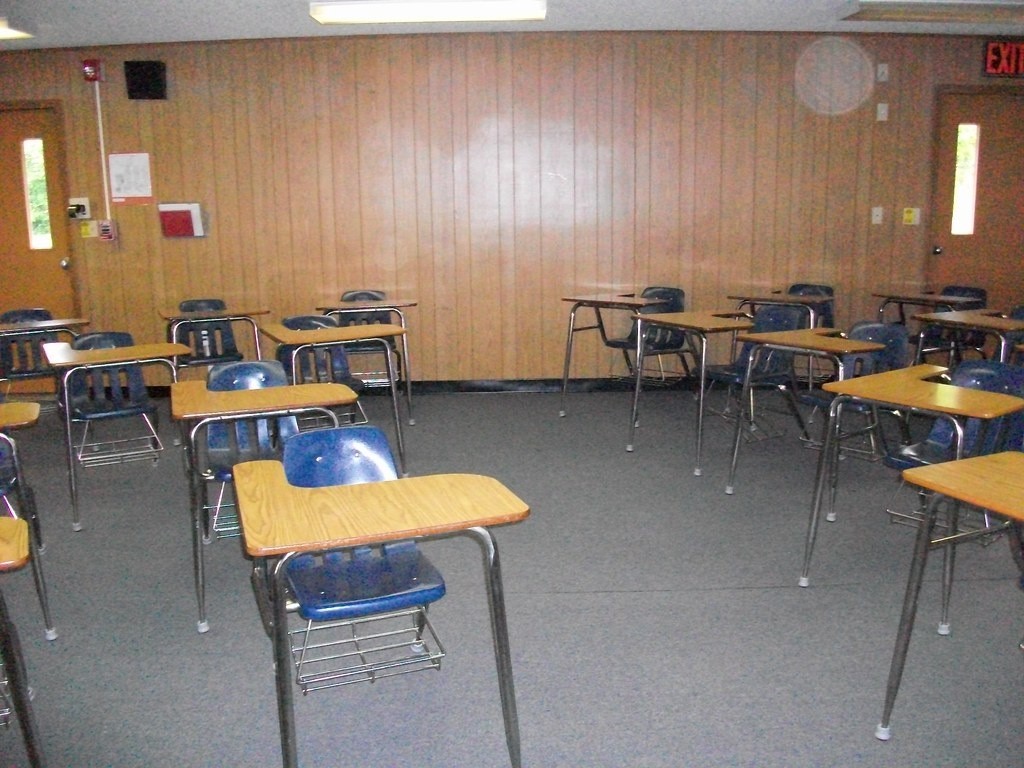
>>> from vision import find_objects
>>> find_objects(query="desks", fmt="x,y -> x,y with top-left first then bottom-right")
43,343 -> 194,532
724,329 -> 886,522
229,459 -> 531,767
0,516 -> 42,768
632,312 -> 753,477
558,293 -> 668,418
870,448 -> 1023,742
798,363 -> 1023,587
168,379 -> 358,635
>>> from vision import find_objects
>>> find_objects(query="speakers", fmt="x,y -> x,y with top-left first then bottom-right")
123,60 -> 166,100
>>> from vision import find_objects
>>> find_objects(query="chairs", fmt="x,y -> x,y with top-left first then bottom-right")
0,285 -> 422,533
0,433 -> 58,642
282,426 -> 444,696
608,281 -> 1020,635
